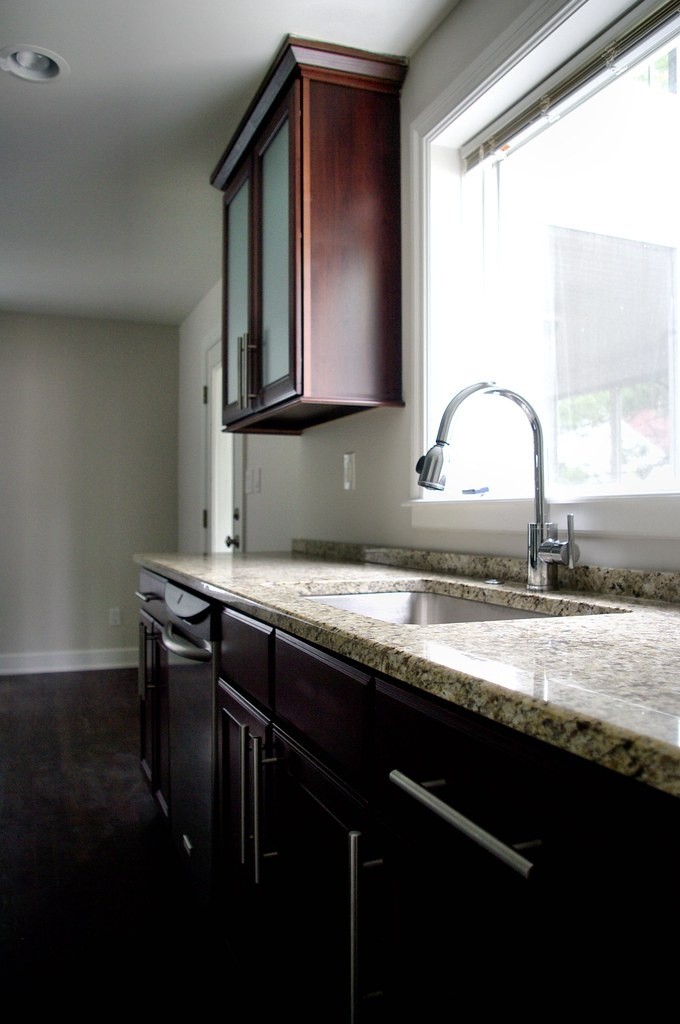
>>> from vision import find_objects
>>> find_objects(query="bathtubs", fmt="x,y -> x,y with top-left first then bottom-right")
0,644 -> 231,883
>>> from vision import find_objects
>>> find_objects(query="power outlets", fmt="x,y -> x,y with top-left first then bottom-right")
109,608 -> 121,627
343,452 -> 356,491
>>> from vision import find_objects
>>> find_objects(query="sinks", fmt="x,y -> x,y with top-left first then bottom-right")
277,577 -> 634,627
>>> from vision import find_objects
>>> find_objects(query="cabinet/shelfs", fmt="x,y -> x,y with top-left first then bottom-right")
210,34 -> 409,435
134,567 -> 220,873
347,670 -> 680,1024
217,605 -> 374,1024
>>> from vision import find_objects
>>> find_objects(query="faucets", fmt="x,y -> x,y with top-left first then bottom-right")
416,380 -> 581,592
